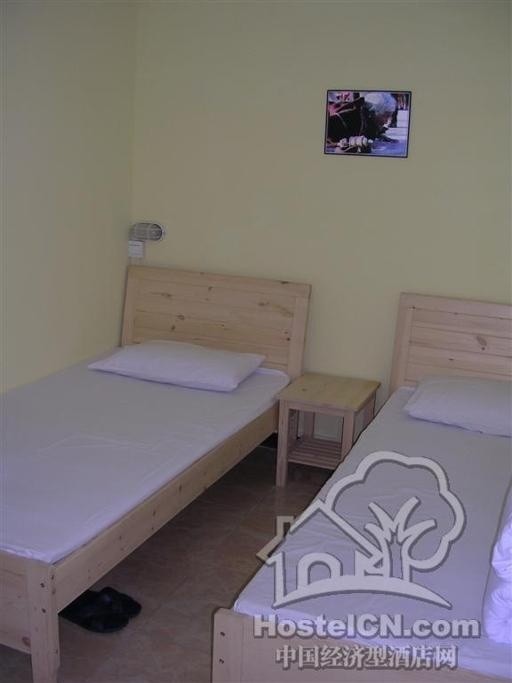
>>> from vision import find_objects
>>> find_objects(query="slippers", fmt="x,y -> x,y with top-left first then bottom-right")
58,596 -> 131,633
83,585 -> 142,618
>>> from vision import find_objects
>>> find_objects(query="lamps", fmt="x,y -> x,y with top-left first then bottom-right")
128,220 -> 165,243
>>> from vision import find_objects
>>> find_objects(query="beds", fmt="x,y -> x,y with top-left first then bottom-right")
1,261 -> 313,683
210,291 -> 511,683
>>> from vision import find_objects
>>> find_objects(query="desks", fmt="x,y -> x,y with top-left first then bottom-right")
272,371 -> 383,486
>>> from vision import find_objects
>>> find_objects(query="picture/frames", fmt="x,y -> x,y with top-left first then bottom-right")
323,86 -> 411,159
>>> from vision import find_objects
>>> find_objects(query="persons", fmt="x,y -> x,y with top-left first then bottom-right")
328,91 -> 400,153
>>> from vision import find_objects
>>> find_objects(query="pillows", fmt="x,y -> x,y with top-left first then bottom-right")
89,337 -> 269,393
402,373 -> 512,438
